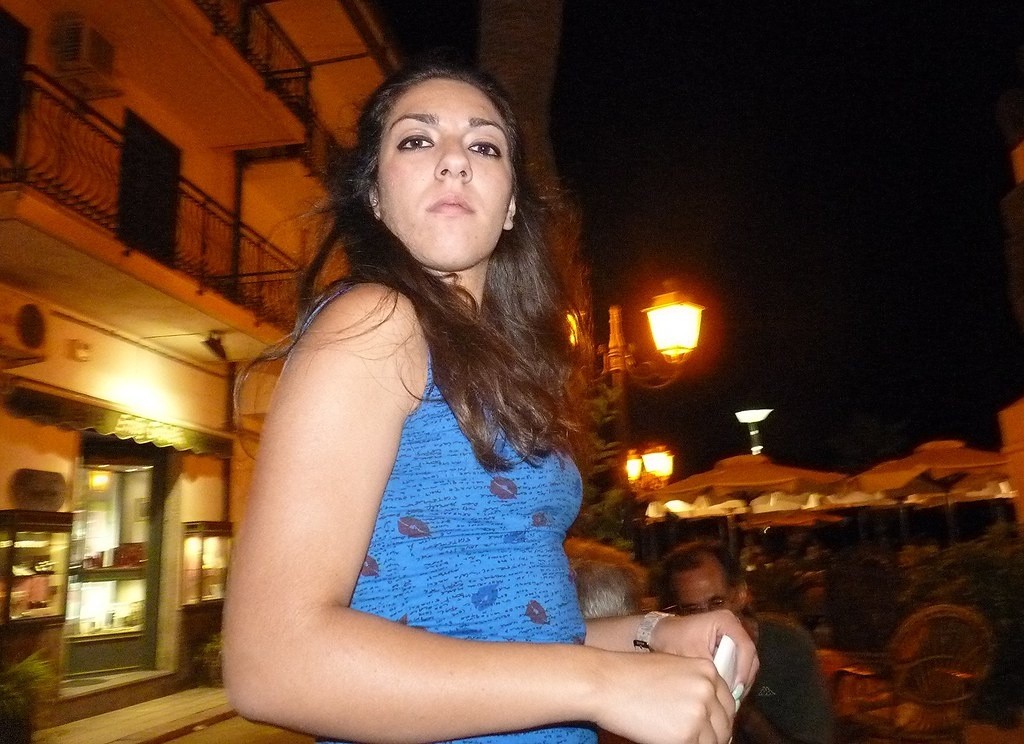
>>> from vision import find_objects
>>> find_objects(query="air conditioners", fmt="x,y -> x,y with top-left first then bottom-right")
55,18 -> 118,96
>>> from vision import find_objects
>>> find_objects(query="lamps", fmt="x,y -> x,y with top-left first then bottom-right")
623,276 -> 709,393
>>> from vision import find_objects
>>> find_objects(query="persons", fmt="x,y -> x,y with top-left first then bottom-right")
562,538 -> 641,620
666,537 -> 836,744
215,69 -> 765,744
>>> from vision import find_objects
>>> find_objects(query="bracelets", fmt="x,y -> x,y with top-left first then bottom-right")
634,611 -> 674,656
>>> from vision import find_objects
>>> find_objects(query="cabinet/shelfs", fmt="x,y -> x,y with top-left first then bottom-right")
0,508 -> 74,631
180,520 -> 235,610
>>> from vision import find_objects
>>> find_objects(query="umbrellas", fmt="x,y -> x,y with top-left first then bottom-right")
849,439 -> 1011,553
667,452 -> 852,564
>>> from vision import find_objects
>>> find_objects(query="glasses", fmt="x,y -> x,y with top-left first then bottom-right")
678,592 -> 740,618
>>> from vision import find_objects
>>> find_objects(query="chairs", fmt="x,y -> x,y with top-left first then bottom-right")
827,603 -> 994,744
